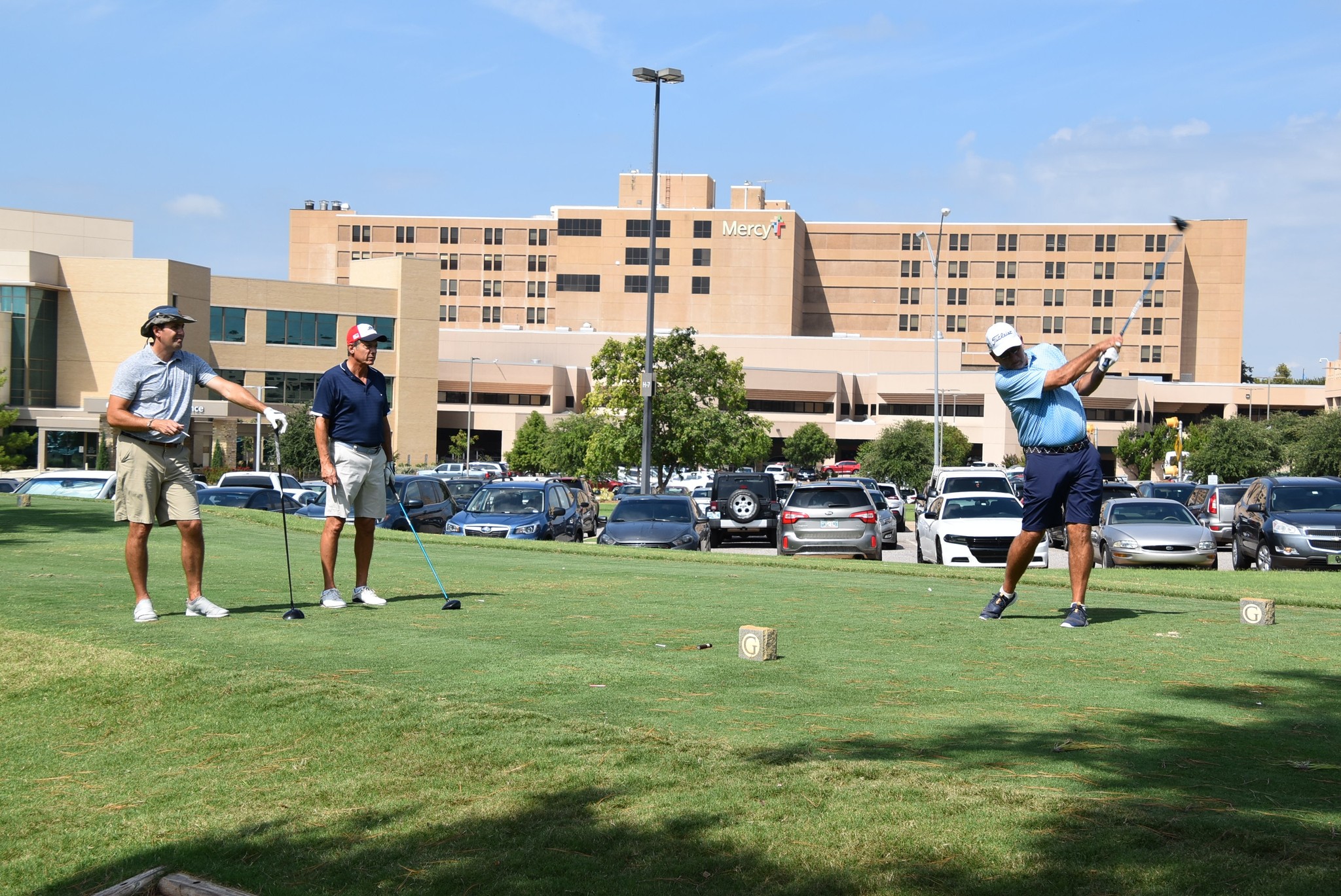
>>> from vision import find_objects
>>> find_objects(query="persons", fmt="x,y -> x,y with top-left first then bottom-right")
105,305 -> 289,622
309,323 -> 398,609
979,321 -> 1124,628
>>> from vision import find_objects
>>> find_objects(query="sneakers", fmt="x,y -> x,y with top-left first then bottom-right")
1060,602 -> 1088,627
979,591 -> 1018,620
134,599 -> 158,622
352,586 -> 387,606
186,596 -> 230,617
320,588 -> 347,608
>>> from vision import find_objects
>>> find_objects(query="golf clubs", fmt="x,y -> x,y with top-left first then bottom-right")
389,478 -> 461,610
1102,216 -> 1190,368
274,420 -> 305,620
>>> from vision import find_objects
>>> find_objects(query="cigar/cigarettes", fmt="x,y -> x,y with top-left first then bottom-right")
180,430 -> 190,437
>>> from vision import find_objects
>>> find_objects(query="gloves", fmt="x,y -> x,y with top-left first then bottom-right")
384,461 -> 395,488
1097,342 -> 1121,372
263,407 -> 288,434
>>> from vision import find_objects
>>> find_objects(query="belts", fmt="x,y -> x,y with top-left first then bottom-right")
121,430 -> 182,448
1025,434 -> 1089,453
332,437 -> 382,454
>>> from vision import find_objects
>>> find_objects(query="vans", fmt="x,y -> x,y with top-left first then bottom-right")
217,471 -> 312,490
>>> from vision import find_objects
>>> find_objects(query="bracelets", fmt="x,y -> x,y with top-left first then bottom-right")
147,417 -> 155,429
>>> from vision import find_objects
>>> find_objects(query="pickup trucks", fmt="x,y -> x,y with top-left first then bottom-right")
415,462 -> 490,479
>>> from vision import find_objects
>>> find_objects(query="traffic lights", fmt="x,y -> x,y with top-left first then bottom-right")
1086,424 -> 1095,434
1165,417 -> 1180,429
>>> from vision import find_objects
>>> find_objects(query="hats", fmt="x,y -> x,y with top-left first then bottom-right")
347,323 -> 387,344
985,322 -> 1022,356
141,305 -> 197,338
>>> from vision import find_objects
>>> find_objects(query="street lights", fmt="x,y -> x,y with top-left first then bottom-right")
1238,376 -> 1286,421
465,357 -> 481,477
915,207 -> 954,467
241,383 -> 278,471
633,66 -> 685,496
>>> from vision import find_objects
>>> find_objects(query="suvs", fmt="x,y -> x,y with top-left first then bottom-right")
294,475 -> 491,534
445,477 -> 599,543
769,477 -> 918,561
1237,477 -> 1259,485
1232,476 -> 1341,572
1136,480 -> 1197,505
1046,476 -> 1138,552
1168,473 -> 1193,483
821,460 -> 860,476
970,461 -> 998,468
704,471 -> 784,547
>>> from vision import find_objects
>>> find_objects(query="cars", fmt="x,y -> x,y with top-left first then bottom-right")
469,461 -> 524,476
528,462 -> 819,501
0,466 -> 117,501
1007,464 -> 1025,506
195,481 -> 321,514
597,495 -> 710,552
1091,497 -> 1218,570
916,490 -> 1049,569
1185,483 -> 1251,546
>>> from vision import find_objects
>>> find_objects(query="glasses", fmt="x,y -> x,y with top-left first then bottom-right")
992,346 -> 1021,359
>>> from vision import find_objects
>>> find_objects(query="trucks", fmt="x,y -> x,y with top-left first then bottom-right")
1161,451 -> 1190,479
915,467 -> 1015,545
